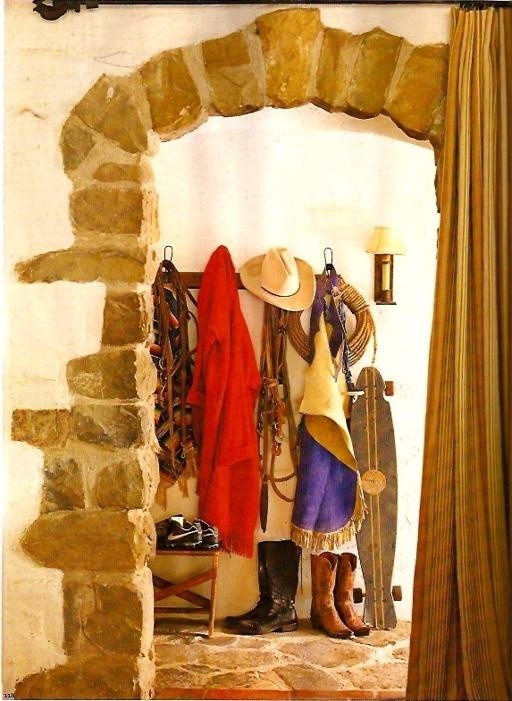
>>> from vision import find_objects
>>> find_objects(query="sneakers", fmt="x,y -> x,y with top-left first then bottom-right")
155,514 -> 218,549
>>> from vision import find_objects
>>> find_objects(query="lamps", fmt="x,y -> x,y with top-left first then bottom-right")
365,225 -> 405,304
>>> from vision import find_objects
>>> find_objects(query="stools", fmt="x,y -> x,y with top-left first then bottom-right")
153,544 -> 222,639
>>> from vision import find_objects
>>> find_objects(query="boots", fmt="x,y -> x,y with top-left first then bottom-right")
334,552 -> 369,635
310,551 -> 354,638
225,540 -> 301,635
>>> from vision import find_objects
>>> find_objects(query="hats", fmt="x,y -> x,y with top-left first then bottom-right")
240,248 -> 316,311
